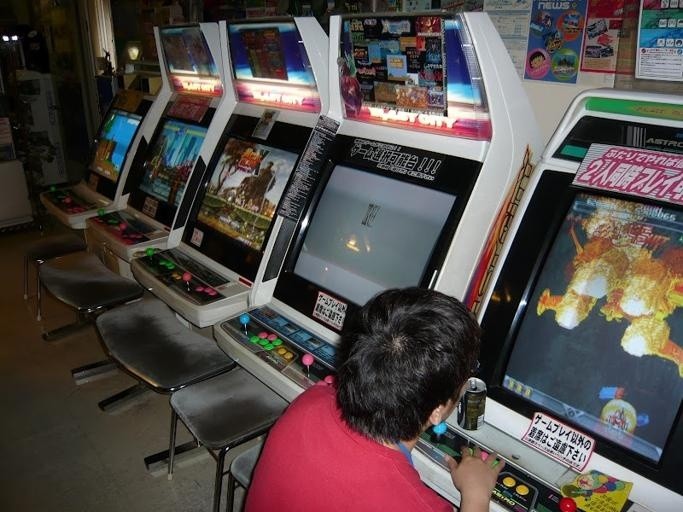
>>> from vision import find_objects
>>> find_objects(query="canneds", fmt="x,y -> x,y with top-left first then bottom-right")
458,377 -> 488,430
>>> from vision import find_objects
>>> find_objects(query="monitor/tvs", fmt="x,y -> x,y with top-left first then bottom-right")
476,169 -> 683,496
123,99 -> 217,231
86,94 -> 153,197
273,130 -> 480,355
189,113 -> 313,285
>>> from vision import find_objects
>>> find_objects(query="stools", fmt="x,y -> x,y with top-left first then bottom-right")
97,299 -> 237,392
168,367 -> 294,511
226,440 -> 265,510
23,249 -> 146,323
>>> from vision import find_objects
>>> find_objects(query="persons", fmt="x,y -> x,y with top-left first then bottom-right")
246,285 -> 507,511
